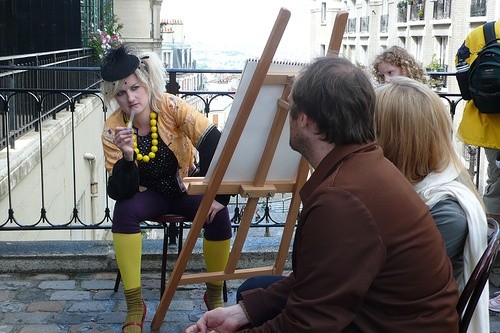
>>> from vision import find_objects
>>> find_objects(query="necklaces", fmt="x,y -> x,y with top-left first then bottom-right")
126,111 -> 161,163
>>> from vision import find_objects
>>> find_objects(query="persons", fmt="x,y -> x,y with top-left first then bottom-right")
94,48 -> 233,333
179,22 -> 500,333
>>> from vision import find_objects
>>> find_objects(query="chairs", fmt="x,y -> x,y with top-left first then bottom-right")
458,216 -> 500,333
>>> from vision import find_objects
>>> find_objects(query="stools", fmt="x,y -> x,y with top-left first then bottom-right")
111,213 -> 228,303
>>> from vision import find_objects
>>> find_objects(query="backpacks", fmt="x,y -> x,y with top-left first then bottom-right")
468,20 -> 500,113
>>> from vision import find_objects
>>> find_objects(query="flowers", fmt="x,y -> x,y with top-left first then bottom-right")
86,12 -> 124,63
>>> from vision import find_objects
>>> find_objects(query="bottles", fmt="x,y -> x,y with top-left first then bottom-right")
455,59 -> 470,72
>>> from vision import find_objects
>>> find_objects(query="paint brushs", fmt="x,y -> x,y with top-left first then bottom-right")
127,109 -> 136,130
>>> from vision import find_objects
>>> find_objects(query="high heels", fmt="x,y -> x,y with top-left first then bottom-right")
122,301 -> 148,333
204,290 -> 226,312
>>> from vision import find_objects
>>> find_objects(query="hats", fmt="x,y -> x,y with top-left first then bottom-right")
100,49 -> 140,82
